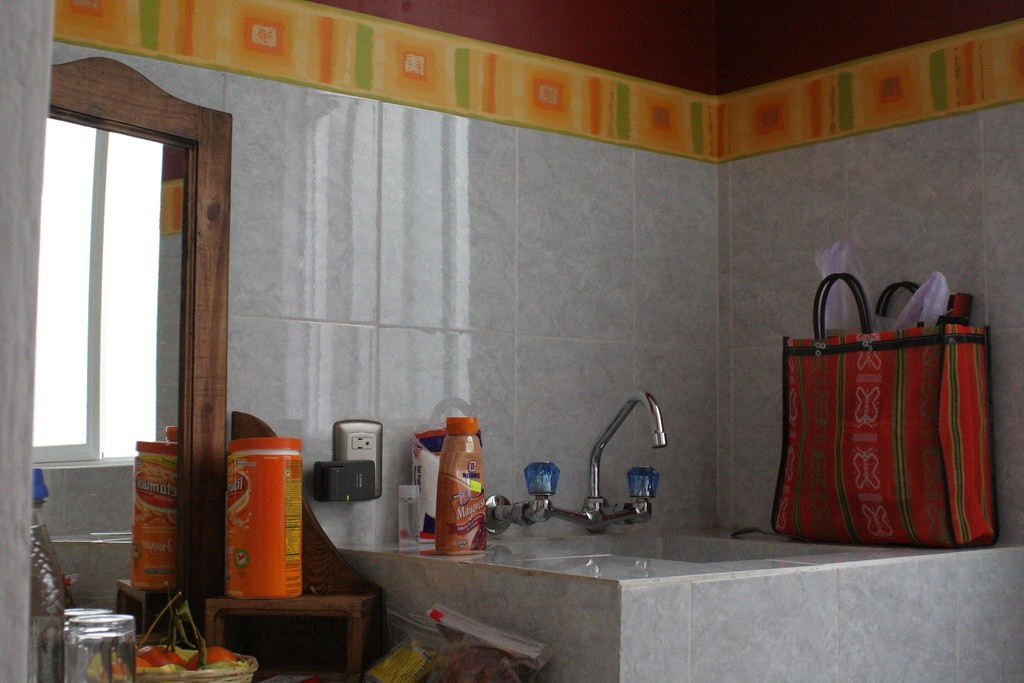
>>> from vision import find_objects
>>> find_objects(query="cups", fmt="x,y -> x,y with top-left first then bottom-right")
65,614 -> 137,683
63,608 -> 114,678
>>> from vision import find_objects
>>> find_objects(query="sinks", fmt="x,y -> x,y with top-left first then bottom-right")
465,521 -> 857,587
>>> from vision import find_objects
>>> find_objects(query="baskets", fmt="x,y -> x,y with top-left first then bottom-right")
97,611 -> 259,683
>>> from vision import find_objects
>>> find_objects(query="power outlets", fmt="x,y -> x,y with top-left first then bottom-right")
333,420 -> 383,499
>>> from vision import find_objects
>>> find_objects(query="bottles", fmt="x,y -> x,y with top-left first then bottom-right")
27,467 -> 65,683
435,418 -> 488,556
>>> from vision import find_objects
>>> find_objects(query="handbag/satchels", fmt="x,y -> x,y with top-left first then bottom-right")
771,272 -> 1000,549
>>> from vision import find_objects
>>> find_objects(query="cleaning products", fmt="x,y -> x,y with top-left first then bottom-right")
431,416 -> 489,553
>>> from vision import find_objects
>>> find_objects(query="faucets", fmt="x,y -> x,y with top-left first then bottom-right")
523,388 -> 668,532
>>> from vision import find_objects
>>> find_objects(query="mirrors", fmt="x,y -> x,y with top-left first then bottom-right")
31,57 -> 234,645
